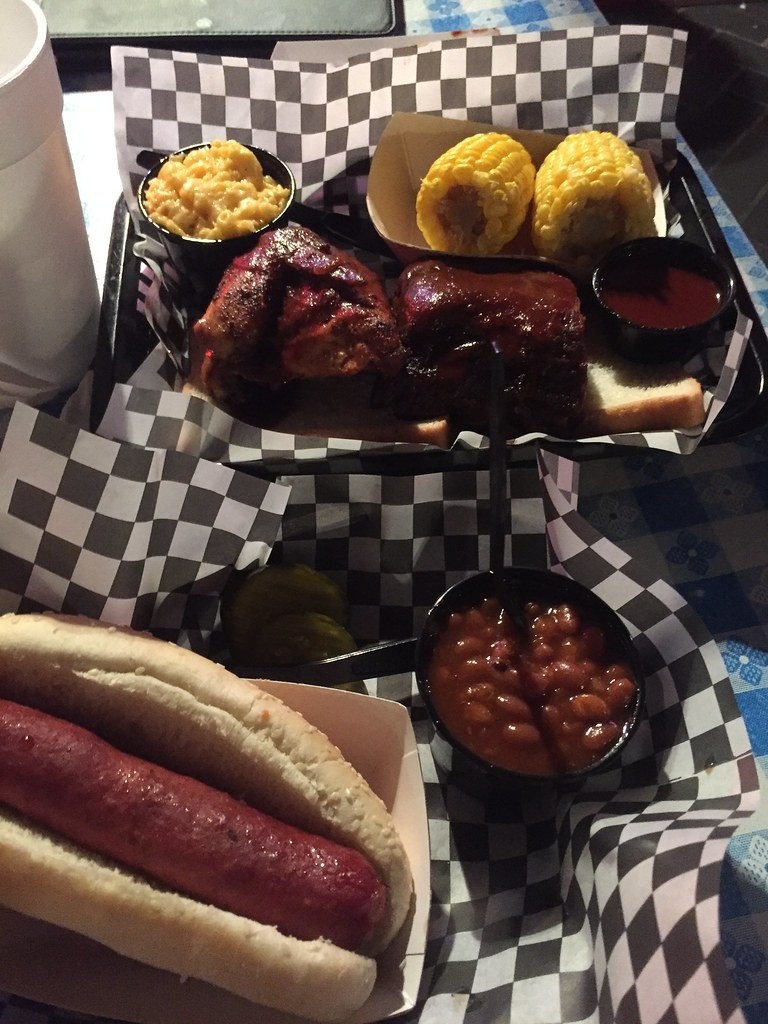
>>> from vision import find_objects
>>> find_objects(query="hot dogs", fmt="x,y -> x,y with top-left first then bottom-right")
1,603 -> 412,1014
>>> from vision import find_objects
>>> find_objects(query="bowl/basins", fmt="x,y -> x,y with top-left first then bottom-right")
417,565 -> 644,784
138,140 -> 297,249
594,238 -> 735,336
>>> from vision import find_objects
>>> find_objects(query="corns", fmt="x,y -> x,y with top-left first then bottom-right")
411,131 -> 658,258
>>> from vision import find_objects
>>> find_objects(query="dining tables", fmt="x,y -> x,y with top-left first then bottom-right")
0,2 -> 768,1023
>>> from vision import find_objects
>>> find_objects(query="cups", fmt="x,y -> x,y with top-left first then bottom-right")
1,0 -> 103,372
